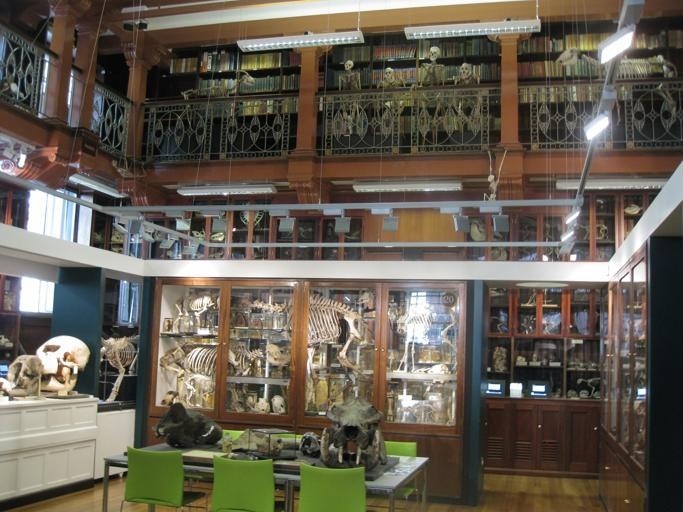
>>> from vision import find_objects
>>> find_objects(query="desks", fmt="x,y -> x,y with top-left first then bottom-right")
100,441 -> 430,511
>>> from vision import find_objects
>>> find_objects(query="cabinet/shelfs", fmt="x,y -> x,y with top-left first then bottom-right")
318,41 -> 500,151
151,278 -> 224,418
221,279 -> 296,418
570,408 -> 599,479
151,50 -> 298,151
485,405 -> 509,470
599,246 -> 648,463
486,288 -> 600,404
509,403 -> 570,475
377,284 -> 457,431
519,27 -> 683,137
596,445 -> 644,509
0,403 -> 100,502
302,284 -> 386,422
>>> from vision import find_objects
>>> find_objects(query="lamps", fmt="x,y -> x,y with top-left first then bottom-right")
236,32 -> 364,52
554,174 -> 668,191
404,20 -> 540,40
586,115 -> 611,145
176,182 -> 280,199
566,209 -> 581,224
353,179 -> 463,193
560,229 -> 574,241
595,23 -> 637,66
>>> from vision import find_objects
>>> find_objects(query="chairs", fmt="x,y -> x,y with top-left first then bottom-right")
296,464 -> 369,512
209,455 -> 286,511
119,447 -> 207,507
362,442 -> 421,509
